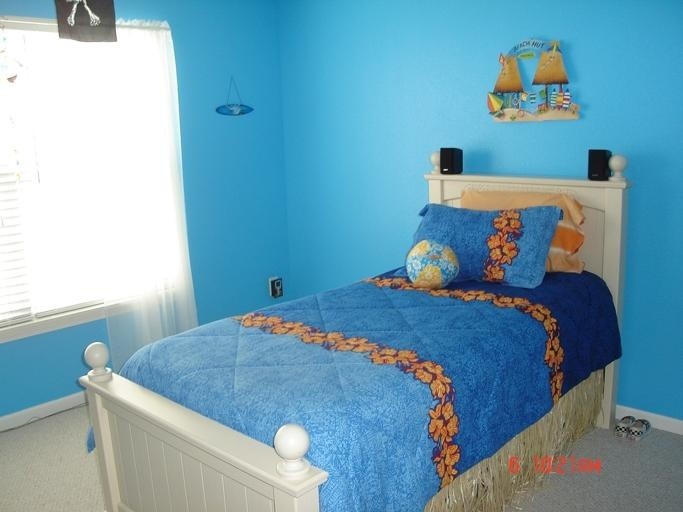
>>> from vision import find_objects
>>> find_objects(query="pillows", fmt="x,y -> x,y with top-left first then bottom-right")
404,185 -> 588,290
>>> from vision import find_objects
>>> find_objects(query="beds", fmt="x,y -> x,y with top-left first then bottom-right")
76,154 -> 628,511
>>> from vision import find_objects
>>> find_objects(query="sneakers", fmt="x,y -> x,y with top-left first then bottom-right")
627,419 -> 651,441
614,416 -> 635,439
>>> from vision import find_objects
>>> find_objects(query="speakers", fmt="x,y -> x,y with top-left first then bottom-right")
588,149 -> 612,181
440,147 -> 463,174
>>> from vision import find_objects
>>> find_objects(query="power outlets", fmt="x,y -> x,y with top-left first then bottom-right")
268,274 -> 283,298
